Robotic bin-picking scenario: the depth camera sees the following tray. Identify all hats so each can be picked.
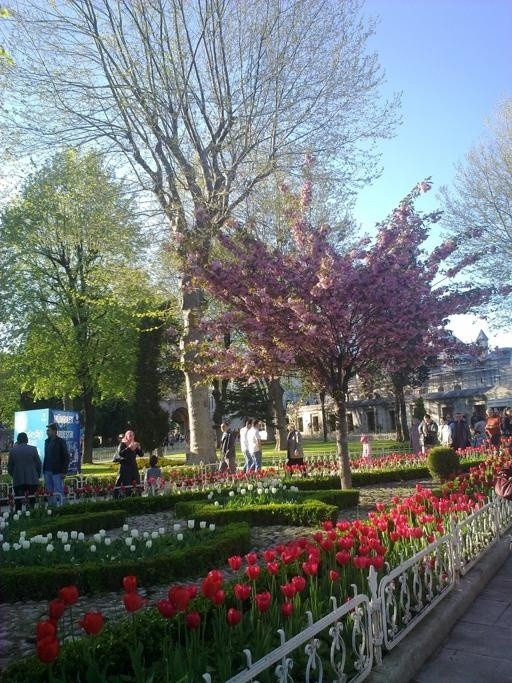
[45,423,57,429]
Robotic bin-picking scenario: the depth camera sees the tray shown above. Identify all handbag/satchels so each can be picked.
[112,450,128,463]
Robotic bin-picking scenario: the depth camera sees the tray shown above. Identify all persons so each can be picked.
[43,423,70,506]
[8,431,42,511]
[284,422,311,478]
[247,419,262,471]
[240,418,254,473]
[360,431,371,458]
[219,421,236,473]
[417,405,511,453]
[113,429,144,497]
